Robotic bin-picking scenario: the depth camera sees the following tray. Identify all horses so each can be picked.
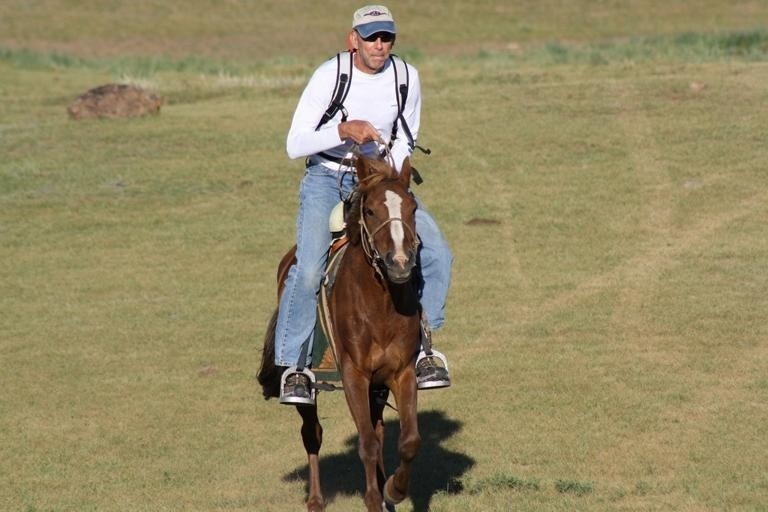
[256,155,423,512]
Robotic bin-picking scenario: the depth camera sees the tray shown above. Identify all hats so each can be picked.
[352,4,396,38]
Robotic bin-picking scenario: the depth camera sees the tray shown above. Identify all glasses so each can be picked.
[362,33,393,42]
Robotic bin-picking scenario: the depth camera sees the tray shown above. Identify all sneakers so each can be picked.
[415,358,448,381]
[285,374,311,397]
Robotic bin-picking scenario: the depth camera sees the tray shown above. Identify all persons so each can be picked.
[274,5,454,396]
